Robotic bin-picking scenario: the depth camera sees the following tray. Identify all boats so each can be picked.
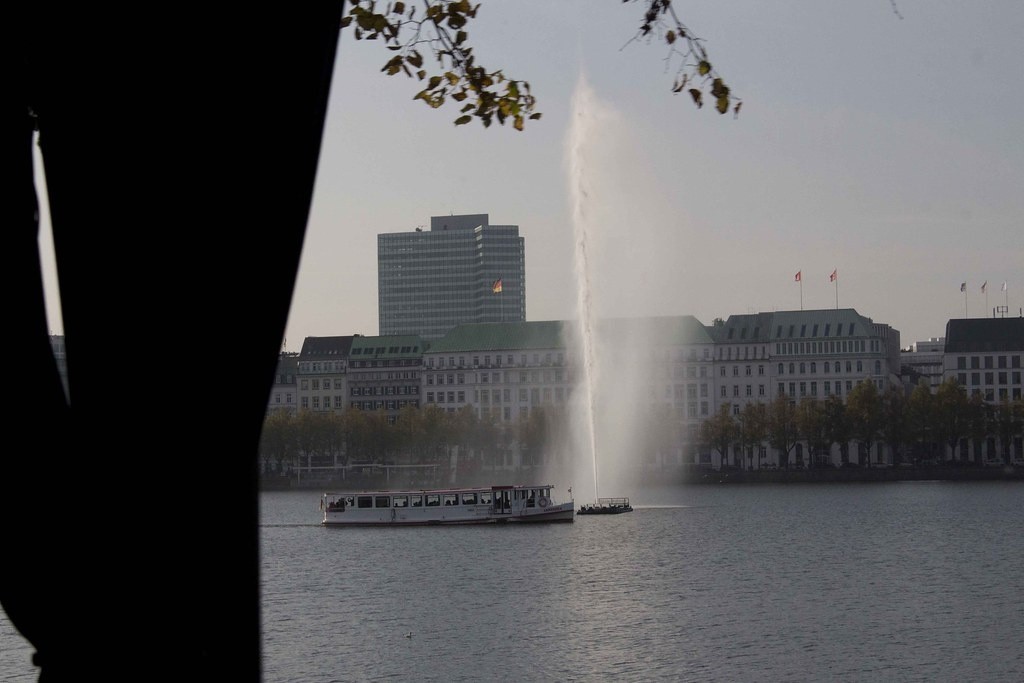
[321,484,575,527]
[577,497,634,516]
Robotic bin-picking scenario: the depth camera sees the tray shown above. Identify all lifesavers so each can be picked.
[538,498,548,507]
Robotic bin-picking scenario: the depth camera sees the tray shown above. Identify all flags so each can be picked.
[959,282,966,296]
[1001,281,1006,290]
[795,271,801,281]
[830,269,836,282]
[981,281,986,293]
[493,278,502,293]
